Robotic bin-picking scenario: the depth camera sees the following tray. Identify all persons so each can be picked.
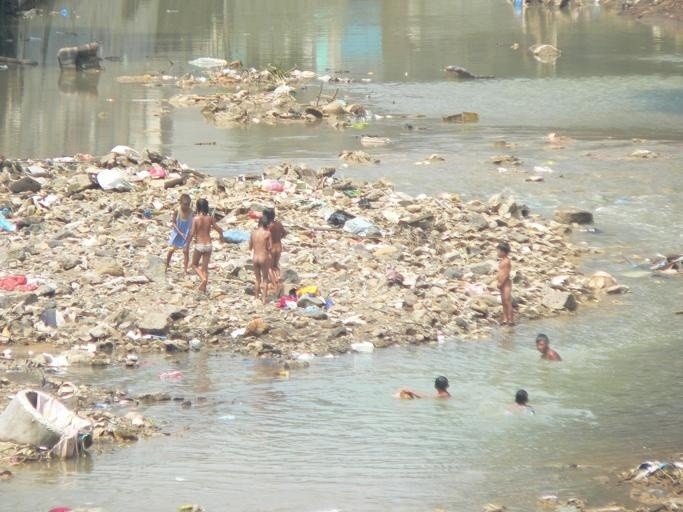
[494,240,516,330]
[261,207,289,288]
[163,194,196,277]
[185,198,224,293]
[534,333,562,363]
[400,376,452,402]
[247,215,273,301]
[510,387,533,411]
